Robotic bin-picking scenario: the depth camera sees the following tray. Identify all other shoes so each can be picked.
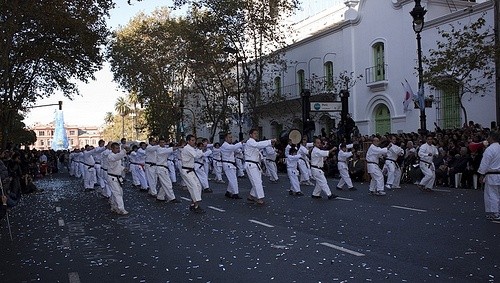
[132,184,163,201]
[337,186,342,190]
[385,185,391,189]
[312,195,322,199]
[86,183,100,192]
[350,187,357,191]
[190,204,206,214]
[328,194,337,200]
[225,191,242,199]
[368,190,386,195]
[102,193,129,214]
[290,190,304,196]
[392,186,401,189]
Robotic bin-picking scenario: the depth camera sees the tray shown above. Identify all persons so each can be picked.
[336,145,358,192]
[417,137,439,191]
[285,140,305,196]
[296,126,362,185]
[361,121,500,186]
[180,134,207,213]
[0,149,68,228]
[366,138,388,196]
[384,136,405,190]
[478,132,500,224]
[67,126,278,202]
[310,138,340,199]
[244,128,277,204]
[107,143,129,215]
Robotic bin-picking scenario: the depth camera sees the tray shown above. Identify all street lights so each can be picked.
[408,0,428,143]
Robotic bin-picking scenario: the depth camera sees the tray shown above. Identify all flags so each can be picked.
[402,79,415,113]
